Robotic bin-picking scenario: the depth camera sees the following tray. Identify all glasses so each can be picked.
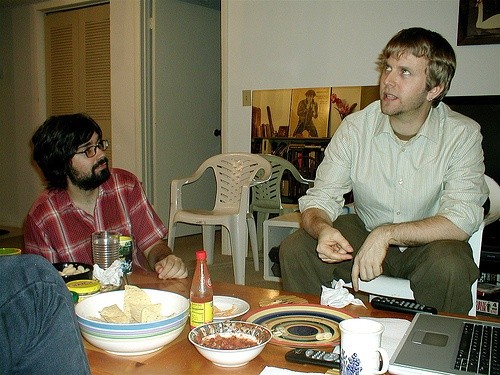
[75,139,109,158]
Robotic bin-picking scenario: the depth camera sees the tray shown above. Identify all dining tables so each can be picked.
[79,273,500,375]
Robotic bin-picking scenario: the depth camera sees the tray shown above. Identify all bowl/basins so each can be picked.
[65,279,100,296]
[74,288,190,355]
[51,261,93,282]
[188,321,273,368]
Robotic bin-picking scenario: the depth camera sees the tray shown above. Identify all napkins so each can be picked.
[320,285,368,308]
[94,260,125,287]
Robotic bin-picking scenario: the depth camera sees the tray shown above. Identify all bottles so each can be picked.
[190,251,213,331]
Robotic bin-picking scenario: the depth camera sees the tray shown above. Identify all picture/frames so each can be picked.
[457,0,500,46]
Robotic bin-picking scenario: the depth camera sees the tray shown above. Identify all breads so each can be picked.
[87,285,176,323]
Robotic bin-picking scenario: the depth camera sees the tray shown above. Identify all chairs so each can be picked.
[233,153,316,261]
[168,152,273,286]
[339,174,500,316]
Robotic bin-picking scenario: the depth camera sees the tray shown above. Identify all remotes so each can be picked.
[284,348,341,369]
[371,297,438,316]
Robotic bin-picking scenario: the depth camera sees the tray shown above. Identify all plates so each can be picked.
[240,304,359,350]
[211,295,251,321]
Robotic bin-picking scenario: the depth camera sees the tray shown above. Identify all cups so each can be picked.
[339,318,390,375]
[118,236,133,276]
[0,247,21,256]
[91,231,120,291]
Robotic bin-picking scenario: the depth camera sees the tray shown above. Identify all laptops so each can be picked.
[389,312,500,375]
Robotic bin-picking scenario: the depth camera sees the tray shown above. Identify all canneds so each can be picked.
[65,280,103,302]
[111,235,133,275]
[0,247,22,256]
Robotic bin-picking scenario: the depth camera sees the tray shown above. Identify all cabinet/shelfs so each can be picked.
[251,136,354,218]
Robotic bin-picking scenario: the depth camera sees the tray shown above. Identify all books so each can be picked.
[258,139,327,200]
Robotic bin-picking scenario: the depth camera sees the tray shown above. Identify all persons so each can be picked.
[0,254,93,375]
[279,28,490,315]
[24,113,190,279]
